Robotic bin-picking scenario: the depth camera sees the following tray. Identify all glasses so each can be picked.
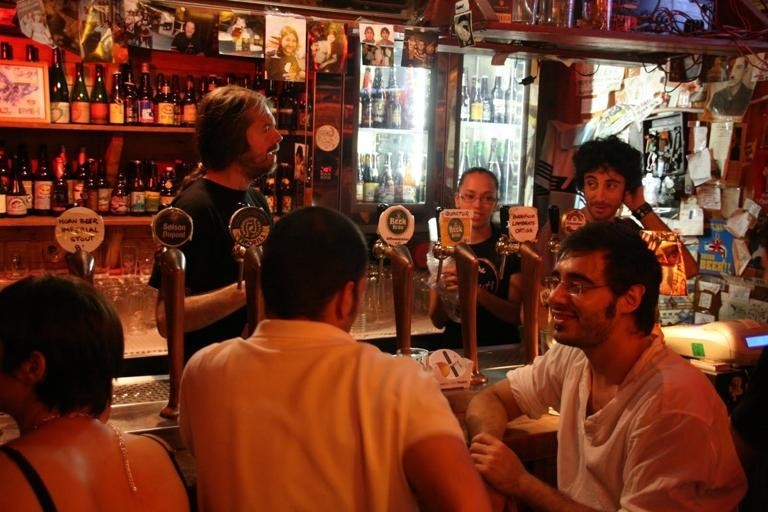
[543,276,609,297]
[457,187,497,205]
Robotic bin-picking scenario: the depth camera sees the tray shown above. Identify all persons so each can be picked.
[464,222,748,511]
[539,137,699,280]
[705,55,753,117]
[147,86,283,368]
[171,21,204,55]
[265,26,305,82]
[729,214,768,512]
[0,272,196,511]
[401,29,438,70]
[83,4,161,63]
[177,204,492,511]
[362,27,395,66]
[309,23,339,73]
[428,168,523,349]
[218,10,264,55]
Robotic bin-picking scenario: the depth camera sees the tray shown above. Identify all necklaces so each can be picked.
[33,411,139,494]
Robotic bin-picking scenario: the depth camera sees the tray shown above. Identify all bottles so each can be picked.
[1,38,302,131]
[353,58,526,206]
[0,140,299,221]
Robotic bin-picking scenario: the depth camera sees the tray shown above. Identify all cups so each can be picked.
[0,240,434,336]
[397,347,430,369]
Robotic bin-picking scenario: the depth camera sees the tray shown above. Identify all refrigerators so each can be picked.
[303,6,545,243]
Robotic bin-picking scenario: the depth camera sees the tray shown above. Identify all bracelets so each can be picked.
[631,203,652,222]
